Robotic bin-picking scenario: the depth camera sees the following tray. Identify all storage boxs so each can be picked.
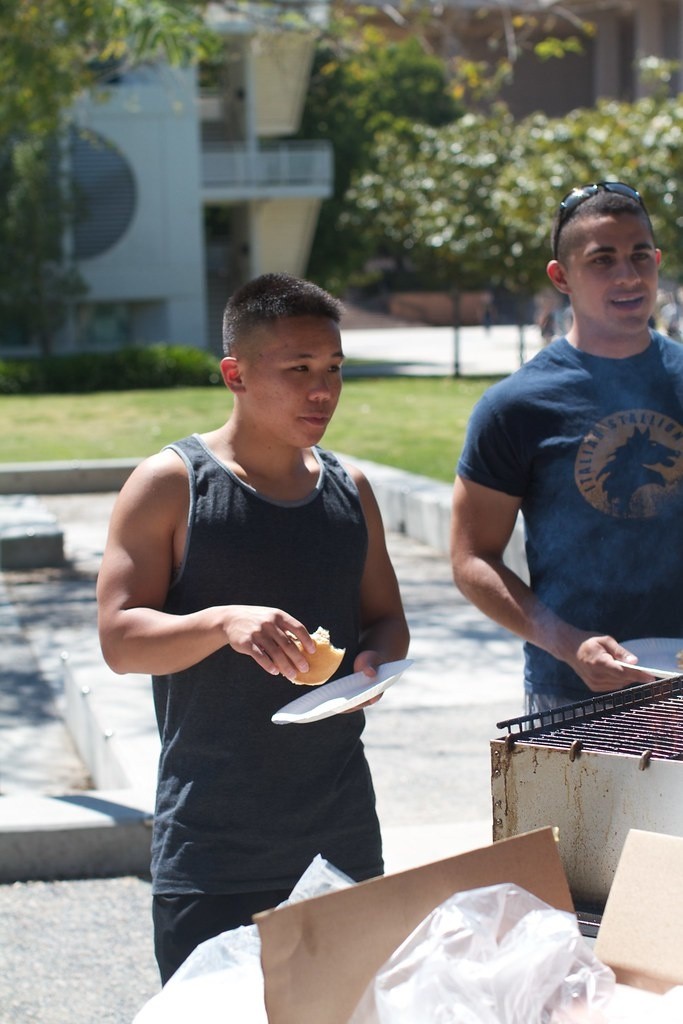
[252,825,683,1023]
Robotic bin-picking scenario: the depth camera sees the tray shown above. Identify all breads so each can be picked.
[283,625,346,686]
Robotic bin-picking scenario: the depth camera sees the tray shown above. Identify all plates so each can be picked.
[270,659,417,724]
[612,638,683,679]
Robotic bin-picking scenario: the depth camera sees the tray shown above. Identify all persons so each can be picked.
[537,296,556,345]
[660,292,683,341]
[97,275,411,985]
[451,185,682,732]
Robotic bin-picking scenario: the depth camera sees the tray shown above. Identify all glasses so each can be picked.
[553,182,649,259]
